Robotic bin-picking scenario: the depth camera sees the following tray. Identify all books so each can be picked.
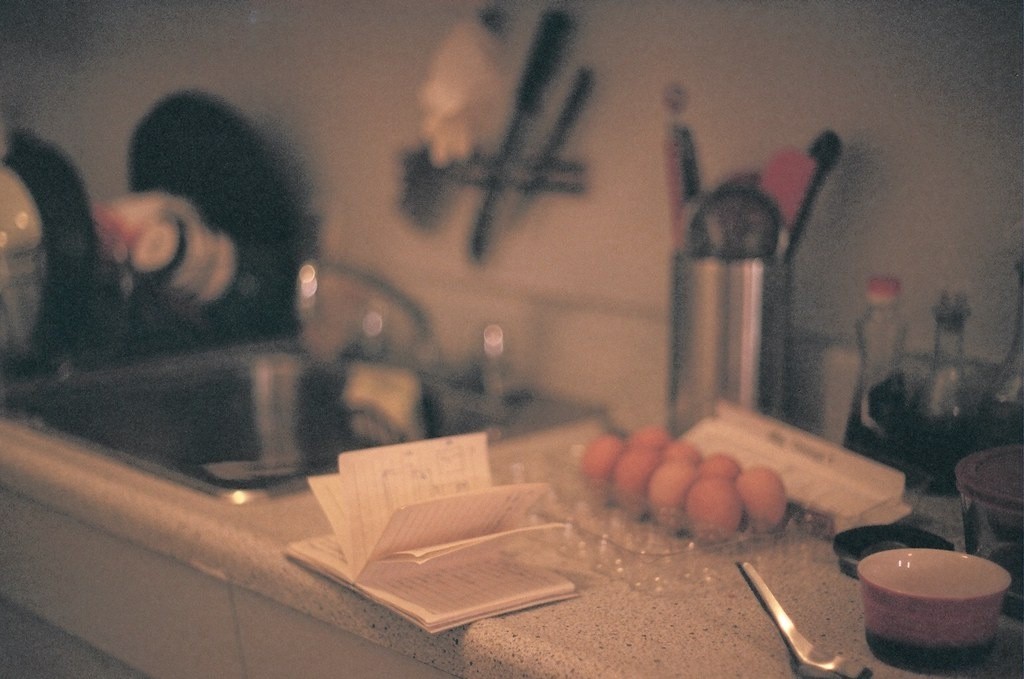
[283,431,582,633]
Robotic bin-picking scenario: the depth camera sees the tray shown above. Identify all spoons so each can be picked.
[736,560,873,679]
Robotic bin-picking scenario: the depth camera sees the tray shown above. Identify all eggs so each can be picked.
[581,425,786,544]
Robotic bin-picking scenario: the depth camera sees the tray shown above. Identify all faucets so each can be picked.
[311,253,438,379]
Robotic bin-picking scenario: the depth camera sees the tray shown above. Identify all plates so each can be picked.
[1,131,93,367]
[126,87,293,243]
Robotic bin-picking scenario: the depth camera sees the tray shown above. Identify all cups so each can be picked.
[670,247,793,439]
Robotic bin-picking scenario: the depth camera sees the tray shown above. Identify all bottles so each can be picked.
[859,259,1023,436]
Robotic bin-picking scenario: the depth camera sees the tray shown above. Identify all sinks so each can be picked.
[0,336,610,503]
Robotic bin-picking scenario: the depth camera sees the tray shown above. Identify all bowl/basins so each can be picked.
[856,547,1012,650]
[954,443,1024,615]
